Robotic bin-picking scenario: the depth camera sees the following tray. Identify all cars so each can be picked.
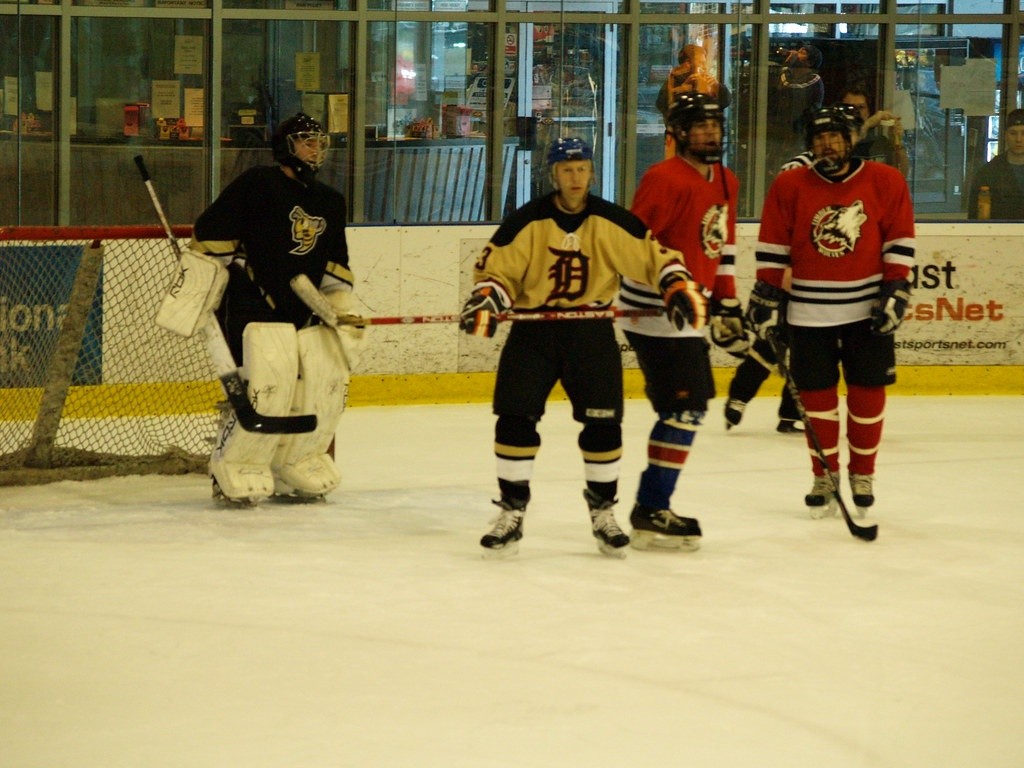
[649,64,674,82]
[918,68,964,126]
[398,21,468,33]
[390,54,417,106]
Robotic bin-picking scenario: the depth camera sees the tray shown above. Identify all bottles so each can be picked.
[977,187,991,219]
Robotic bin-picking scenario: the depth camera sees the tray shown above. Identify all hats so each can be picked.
[803,44,823,69]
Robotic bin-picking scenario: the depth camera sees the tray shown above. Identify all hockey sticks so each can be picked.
[292,271,665,328]
[135,153,318,434]
[766,328,879,540]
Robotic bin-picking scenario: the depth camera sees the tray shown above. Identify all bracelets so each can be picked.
[889,142,906,151]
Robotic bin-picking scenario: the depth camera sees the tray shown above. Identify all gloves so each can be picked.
[708,298,758,353]
[746,281,789,344]
[867,281,912,339]
[658,274,712,332]
[458,286,504,339]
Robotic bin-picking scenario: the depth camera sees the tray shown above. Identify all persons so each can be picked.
[460,136,710,549]
[655,44,733,160]
[723,46,909,428]
[749,110,915,506]
[966,109,1024,222]
[615,91,755,536]
[188,113,366,501]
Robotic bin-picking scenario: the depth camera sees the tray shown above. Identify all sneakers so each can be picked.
[846,469,875,517]
[630,500,703,553]
[777,419,806,436]
[479,498,523,559]
[724,396,746,429]
[211,477,258,509]
[804,473,840,518]
[582,488,630,560]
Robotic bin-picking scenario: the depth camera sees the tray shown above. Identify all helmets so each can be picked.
[272,110,331,188]
[664,89,731,164]
[805,107,853,176]
[547,138,598,192]
